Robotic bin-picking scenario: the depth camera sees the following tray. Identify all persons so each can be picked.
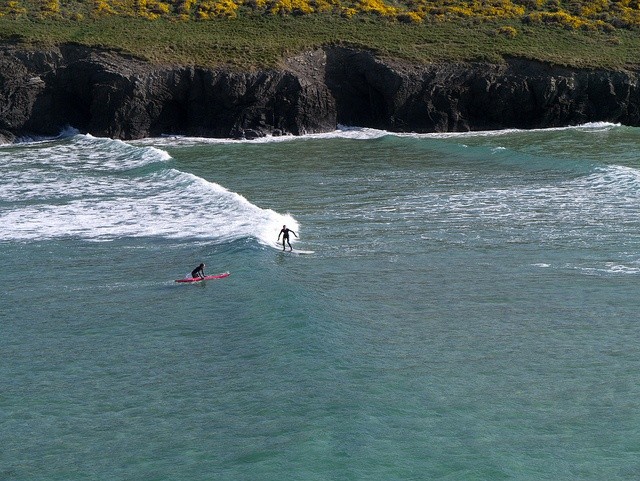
[277,225,297,251]
[192,263,208,279]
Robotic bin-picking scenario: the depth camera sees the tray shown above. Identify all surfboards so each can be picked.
[284,247,315,254]
[175,273,229,282]
